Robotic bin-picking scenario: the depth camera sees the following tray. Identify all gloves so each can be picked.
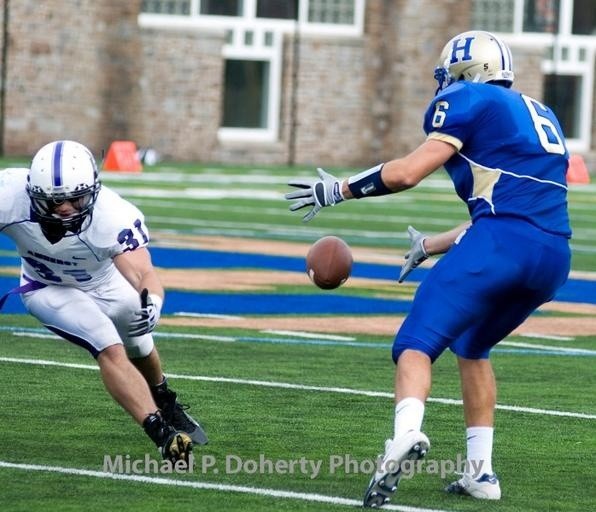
[398,224,431,286]
[284,167,346,224]
[127,286,163,338]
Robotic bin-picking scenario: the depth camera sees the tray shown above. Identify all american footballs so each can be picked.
[305,235,353,290]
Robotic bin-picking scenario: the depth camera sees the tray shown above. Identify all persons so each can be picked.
[285,30,572,509]
[0,140,208,472]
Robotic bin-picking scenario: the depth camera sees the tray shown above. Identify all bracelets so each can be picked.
[149,294,163,309]
[345,164,392,199]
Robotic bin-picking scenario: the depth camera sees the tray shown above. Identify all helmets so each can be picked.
[25,140,101,244]
[433,28,514,95]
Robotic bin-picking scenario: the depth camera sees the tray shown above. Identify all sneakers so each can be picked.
[361,428,431,509]
[156,388,208,445]
[142,411,194,470]
[443,471,503,500]
